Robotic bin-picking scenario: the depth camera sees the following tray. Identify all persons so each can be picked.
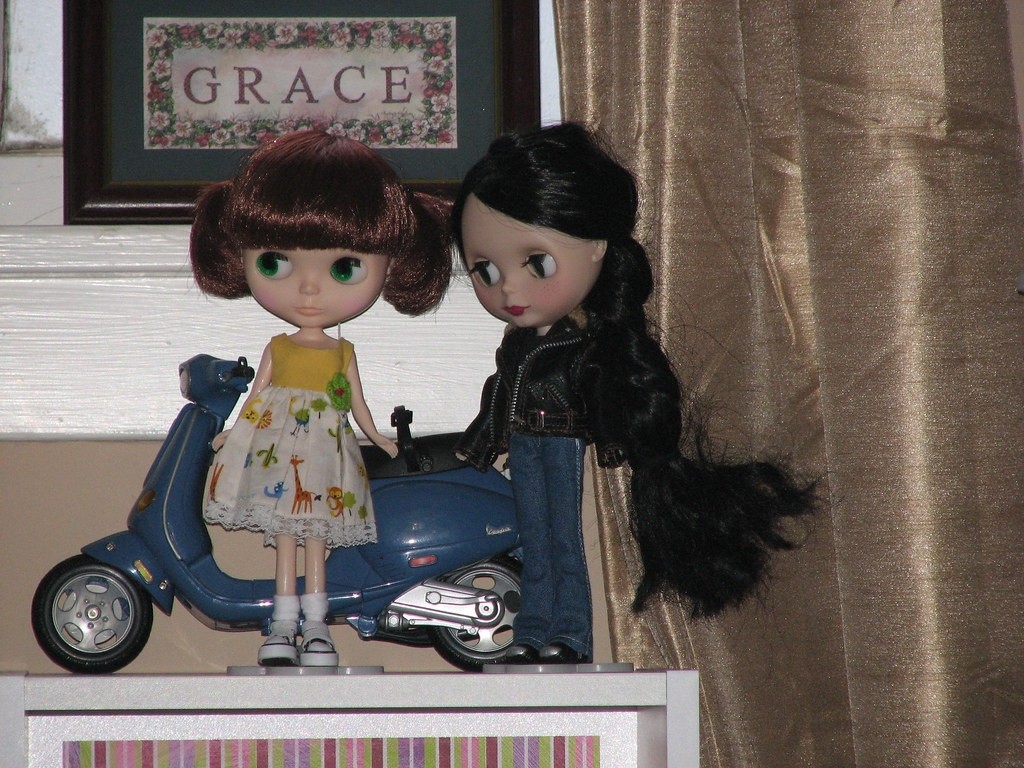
[191,134,456,664]
[449,122,828,664]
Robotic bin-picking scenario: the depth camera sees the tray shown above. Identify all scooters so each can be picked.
[30,353,497,675]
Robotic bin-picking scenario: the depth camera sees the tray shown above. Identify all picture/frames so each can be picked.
[62,0,541,224]
